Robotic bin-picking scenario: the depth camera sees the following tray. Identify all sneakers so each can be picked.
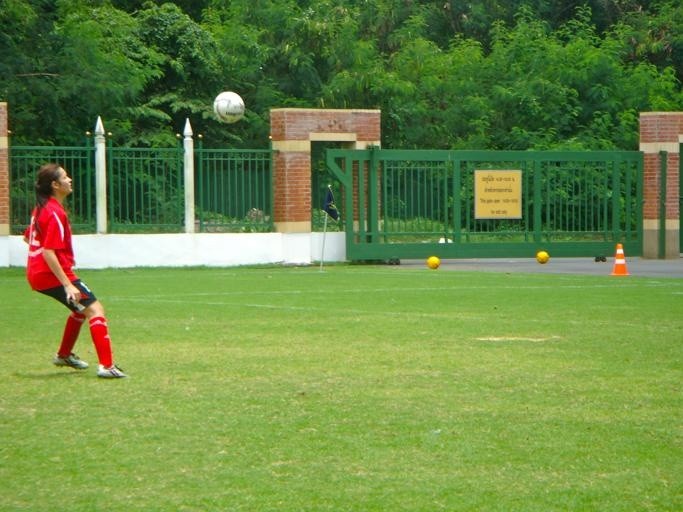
[95,363,129,378]
[52,351,90,369]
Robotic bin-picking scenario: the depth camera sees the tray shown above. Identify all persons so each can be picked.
[20,162,129,378]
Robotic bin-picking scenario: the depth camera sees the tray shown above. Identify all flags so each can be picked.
[321,186,339,221]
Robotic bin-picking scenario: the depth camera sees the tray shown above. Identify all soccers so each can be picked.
[213,91,245,124]
[426,256,440,268]
[537,251,550,264]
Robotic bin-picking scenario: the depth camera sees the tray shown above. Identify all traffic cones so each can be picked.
[608,243,632,276]
